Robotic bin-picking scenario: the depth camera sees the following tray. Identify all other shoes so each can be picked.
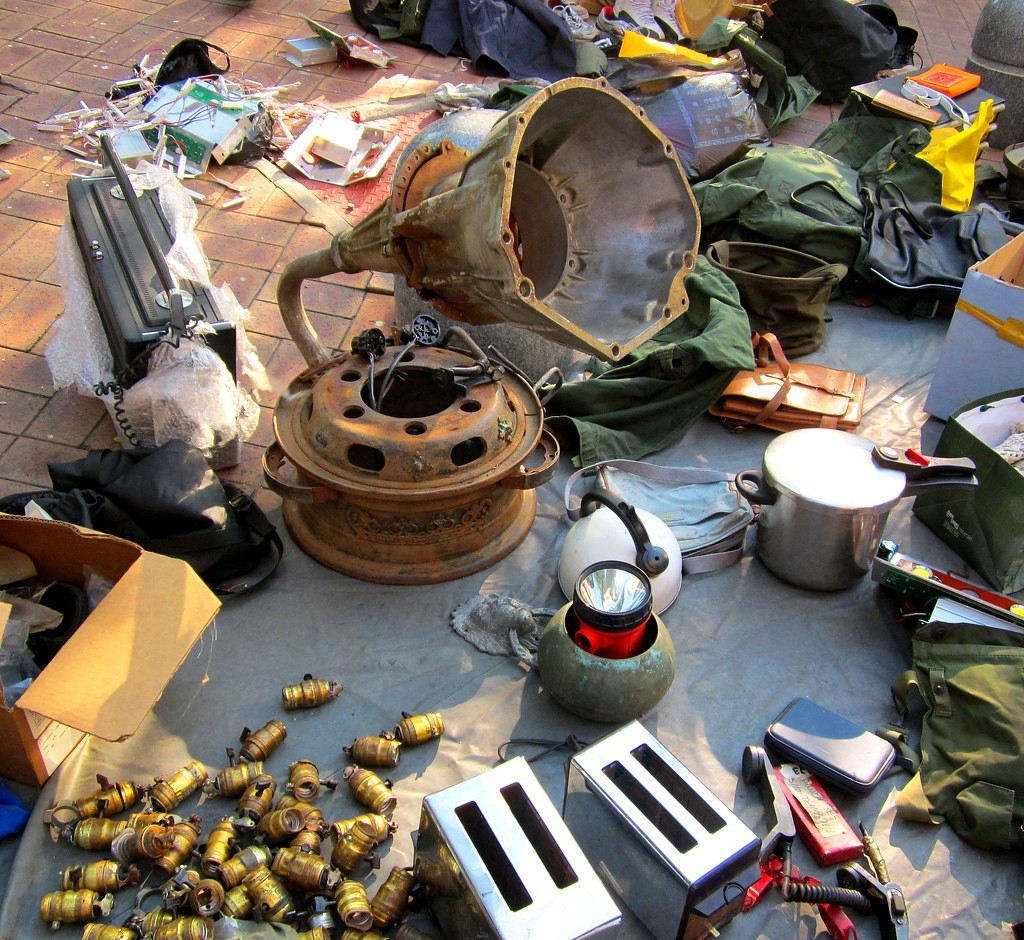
[889,25,924,70]
[561,0,590,20]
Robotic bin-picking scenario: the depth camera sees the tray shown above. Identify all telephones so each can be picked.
[740,741,796,862]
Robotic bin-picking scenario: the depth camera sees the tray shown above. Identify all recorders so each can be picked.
[67,135,242,472]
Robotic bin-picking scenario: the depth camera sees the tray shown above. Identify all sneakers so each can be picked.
[544,0,598,41]
[651,0,691,48]
[613,0,665,40]
[595,5,643,42]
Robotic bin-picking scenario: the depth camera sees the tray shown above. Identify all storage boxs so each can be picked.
[312,113,365,168]
[104,128,153,168]
[923,225,1023,421]
[0,514,223,789]
[284,36,339,69]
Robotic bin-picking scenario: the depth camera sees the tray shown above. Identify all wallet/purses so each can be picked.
[763,696,897,797]
[771,762,865,867]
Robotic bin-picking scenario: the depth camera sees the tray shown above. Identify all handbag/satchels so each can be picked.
[789,178,1007,320]
[706,238,849,361]
[603,54,775,186]
[911,386,1024,595]
[563,456,754,574]
[879,621,1024,853]
[691,15,821,137]
[709,331,870,434]
[1,439,284,599]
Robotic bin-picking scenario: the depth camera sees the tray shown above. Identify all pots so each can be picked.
[736,428,980,591]
